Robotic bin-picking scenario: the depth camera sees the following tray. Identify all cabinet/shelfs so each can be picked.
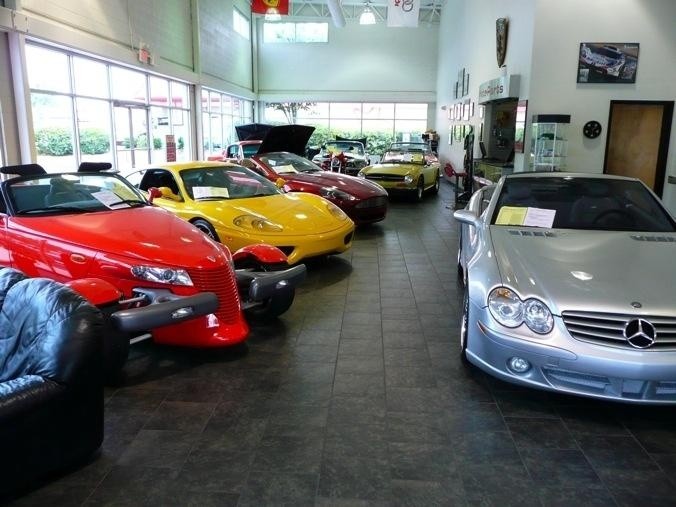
[528,113,571,172]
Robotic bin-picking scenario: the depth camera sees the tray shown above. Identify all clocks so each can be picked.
[583,121,602,138]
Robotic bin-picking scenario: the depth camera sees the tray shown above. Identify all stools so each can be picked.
[445,163,469,210]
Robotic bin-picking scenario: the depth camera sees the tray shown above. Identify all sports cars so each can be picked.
[451,167,676,405]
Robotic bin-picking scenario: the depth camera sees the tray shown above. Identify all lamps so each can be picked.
[360,0,378,26]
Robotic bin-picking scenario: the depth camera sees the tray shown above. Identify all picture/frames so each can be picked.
[576,42,639,83]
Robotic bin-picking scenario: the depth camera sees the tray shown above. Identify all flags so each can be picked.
[386,0,420,28]
[251,0,289,16]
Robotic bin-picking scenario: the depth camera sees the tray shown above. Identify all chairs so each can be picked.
[501,182,539,210]
[571,182,621,228]
[44,178,82,206]
